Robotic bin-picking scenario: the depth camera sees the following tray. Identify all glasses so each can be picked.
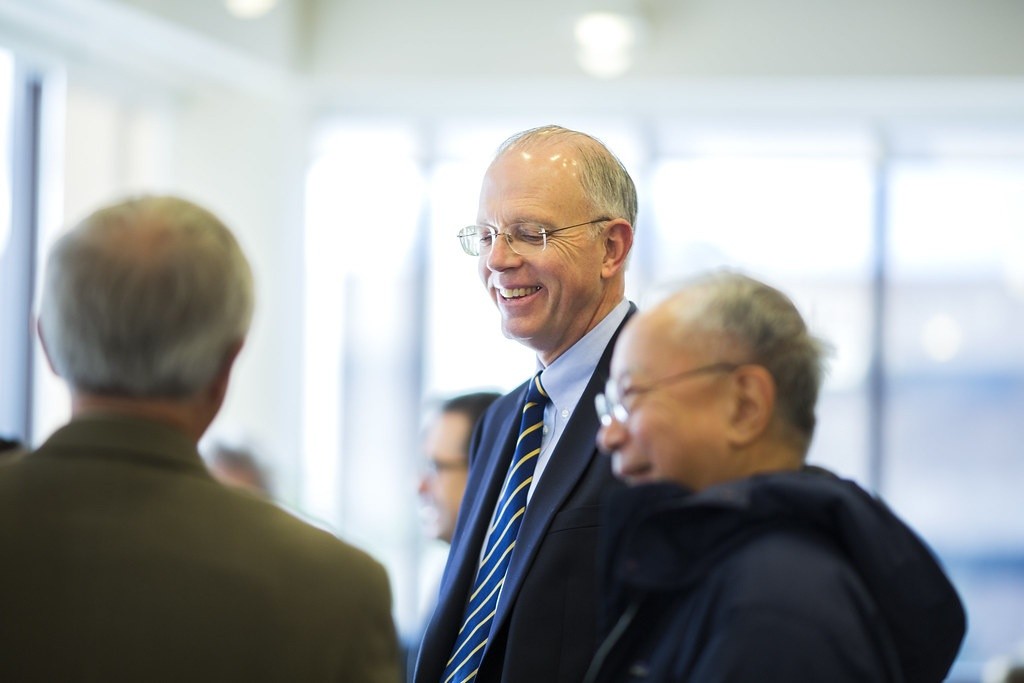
[594,364,740,425]
[458,217,610,261]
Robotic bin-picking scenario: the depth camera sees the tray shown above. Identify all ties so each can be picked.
[440,373,551,683]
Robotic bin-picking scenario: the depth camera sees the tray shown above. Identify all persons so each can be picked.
[417,389,513,548]
[596,270,968,683]
[205,448,278,510]
[403,128,648,683]
[0,199,409,683]
